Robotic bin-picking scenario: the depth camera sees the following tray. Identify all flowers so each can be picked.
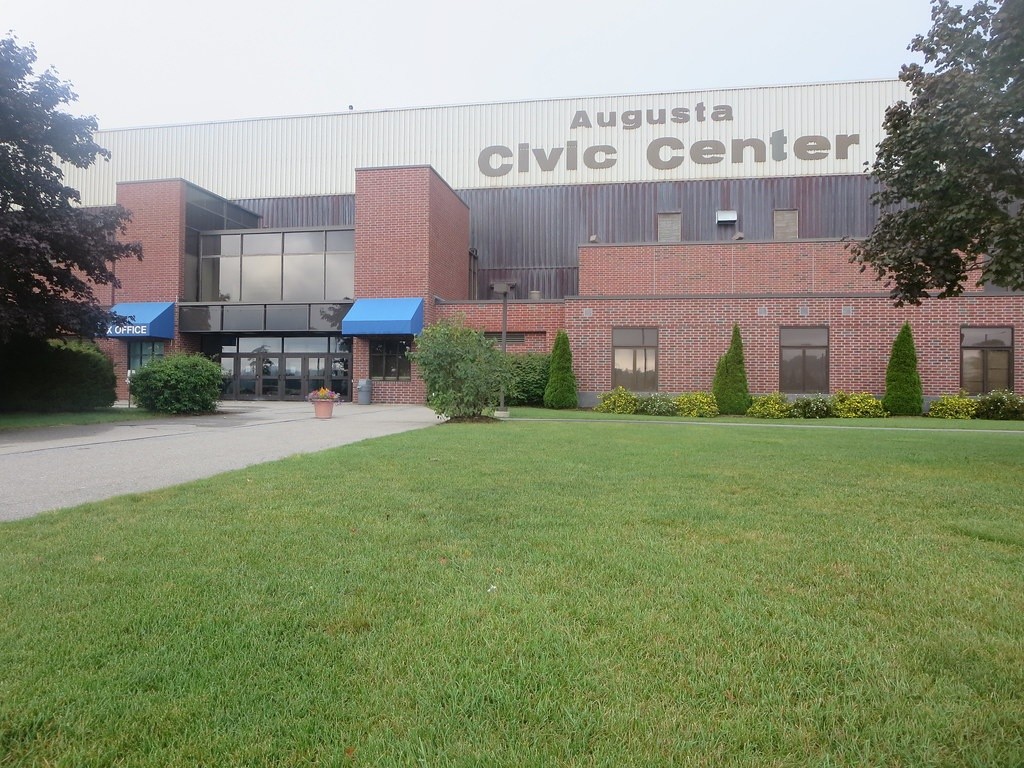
[306,387,344,407]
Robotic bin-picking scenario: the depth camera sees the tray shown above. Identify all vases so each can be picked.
[310,398,334,419]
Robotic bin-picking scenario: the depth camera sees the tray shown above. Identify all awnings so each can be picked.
[342,298,424,335]
[96,302,176,339]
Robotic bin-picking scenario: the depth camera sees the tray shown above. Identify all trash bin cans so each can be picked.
[356,379,372,405]
